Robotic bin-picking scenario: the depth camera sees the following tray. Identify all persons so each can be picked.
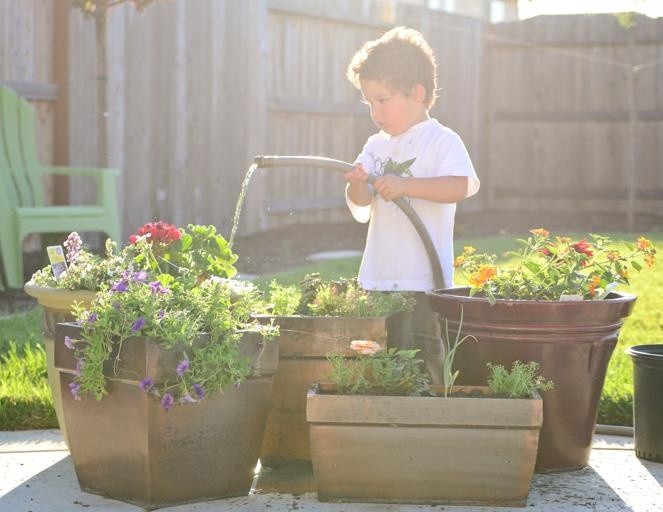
[346,27,481,387]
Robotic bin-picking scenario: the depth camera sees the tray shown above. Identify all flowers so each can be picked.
[452,228,657,303]
[259,272,418,318]
[63,271,280,409]
[322,304,557,397]
[28,218,238,287]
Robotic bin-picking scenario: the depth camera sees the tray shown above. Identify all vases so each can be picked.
[425,285,637,477]
[625,345,663,463]
[22,282,255,454]
[252,312,387,475]
[305,382,544,509]
[52,320,279,511]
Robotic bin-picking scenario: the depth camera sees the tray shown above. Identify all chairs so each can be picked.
[0,83,121,290]
